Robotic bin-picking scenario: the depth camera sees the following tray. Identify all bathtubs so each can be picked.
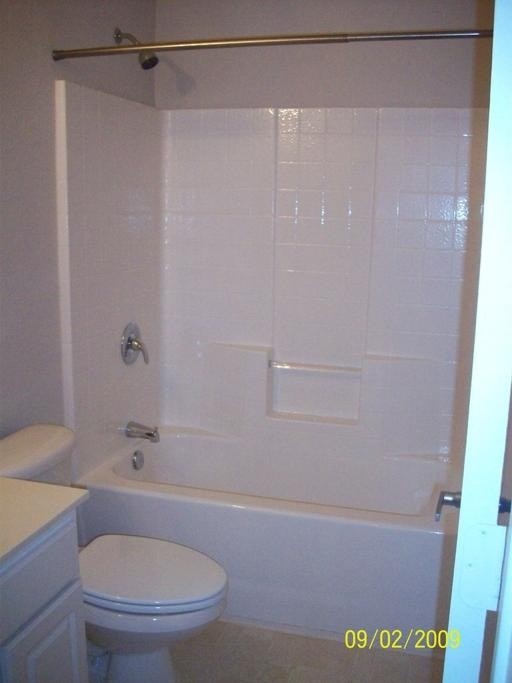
[70,425,464,662]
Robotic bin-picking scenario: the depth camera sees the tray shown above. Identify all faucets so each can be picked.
[124,421,160,443]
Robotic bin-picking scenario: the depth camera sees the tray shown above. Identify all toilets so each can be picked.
[0,421,227,681]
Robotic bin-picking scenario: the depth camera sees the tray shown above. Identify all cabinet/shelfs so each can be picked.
[0,522,93,683]
